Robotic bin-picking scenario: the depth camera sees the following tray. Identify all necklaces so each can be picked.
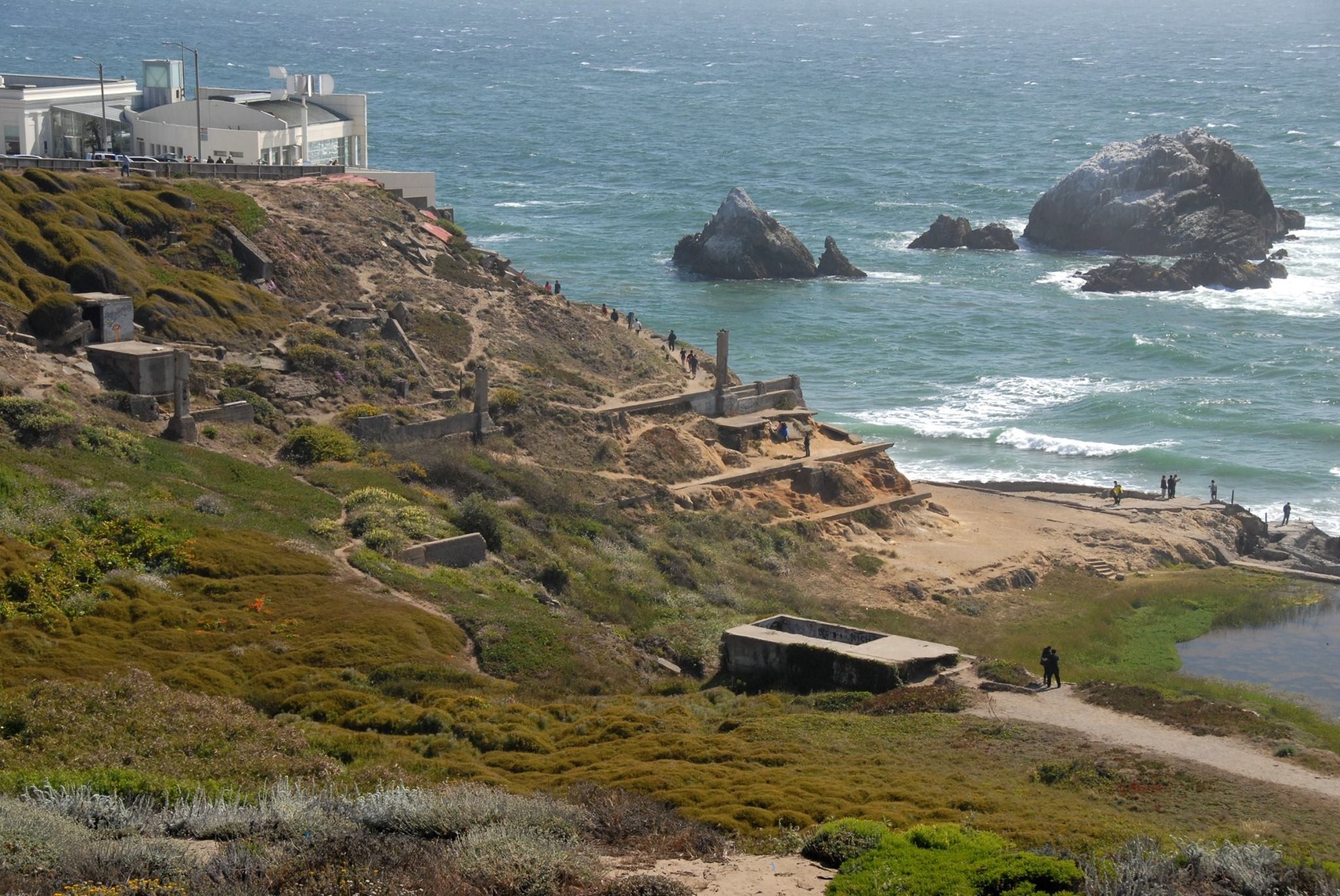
[632,318,641,335]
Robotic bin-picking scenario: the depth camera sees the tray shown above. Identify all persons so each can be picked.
[625,312,636,330]
[1167,474,1180,499]
[1040,645,1062,689]
[327,159,339,166]
[667,330,676,351]
[600,304,608,318]
[691,354,698,380]
[1112,481,1123,508]
[544,281,552,292]
[686,350,694,373]
[1210,480,1217,501]
[1161,475,1167,497]
[120,153,132,177]
[555,280,561,294]
[183,155,234,164]
[680,348,687,367]
[776,419,790,442]
[1282,502,1291,526]
[610,309,619,324]
[803,432,811,458]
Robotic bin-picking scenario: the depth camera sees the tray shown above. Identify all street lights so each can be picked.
[72,56,107,153]
[162,41,201,164]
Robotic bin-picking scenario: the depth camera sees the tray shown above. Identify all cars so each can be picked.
[0,154,42,158]
[92,152,179,162]
